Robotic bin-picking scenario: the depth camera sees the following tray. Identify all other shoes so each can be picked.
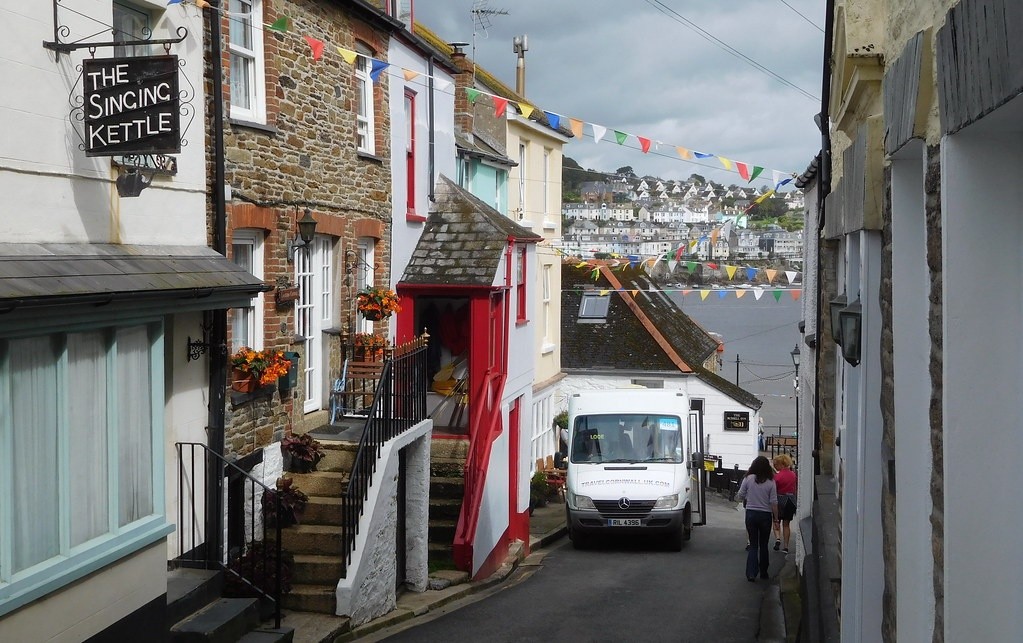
[782,548,788,553]
[761,573,769,578]
[748,576,755,582]
[745,543,750,549]
[774,540,781,550]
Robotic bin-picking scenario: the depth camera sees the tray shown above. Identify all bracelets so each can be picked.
[774,520,779,523]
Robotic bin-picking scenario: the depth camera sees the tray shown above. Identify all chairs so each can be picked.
[537,455,567,504]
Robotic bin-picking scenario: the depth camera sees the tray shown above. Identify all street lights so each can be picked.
[790,342,800,465]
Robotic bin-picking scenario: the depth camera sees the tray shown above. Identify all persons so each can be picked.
[737,456,780,583]
[773,454,798,554]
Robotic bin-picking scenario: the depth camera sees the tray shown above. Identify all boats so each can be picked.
[666,281,802,289]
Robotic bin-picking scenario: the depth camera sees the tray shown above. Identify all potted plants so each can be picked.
[261,475,310,530]
[281,432,326,473]
[225,538,294,619]
[529,472,549,516]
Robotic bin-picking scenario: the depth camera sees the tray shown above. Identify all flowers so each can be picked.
[230,346,292,387]
[352,332,390,358]
[355,285,402,321]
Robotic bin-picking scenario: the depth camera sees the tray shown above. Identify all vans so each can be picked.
[553,388,703,551]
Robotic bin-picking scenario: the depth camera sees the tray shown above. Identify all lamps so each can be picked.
[837,290,862,367]
[827,289,847,347]
[287,199,318,263]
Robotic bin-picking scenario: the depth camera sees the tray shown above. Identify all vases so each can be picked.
[365,310,378,320]
[232,368,256,393]
[354,356,372,363]
